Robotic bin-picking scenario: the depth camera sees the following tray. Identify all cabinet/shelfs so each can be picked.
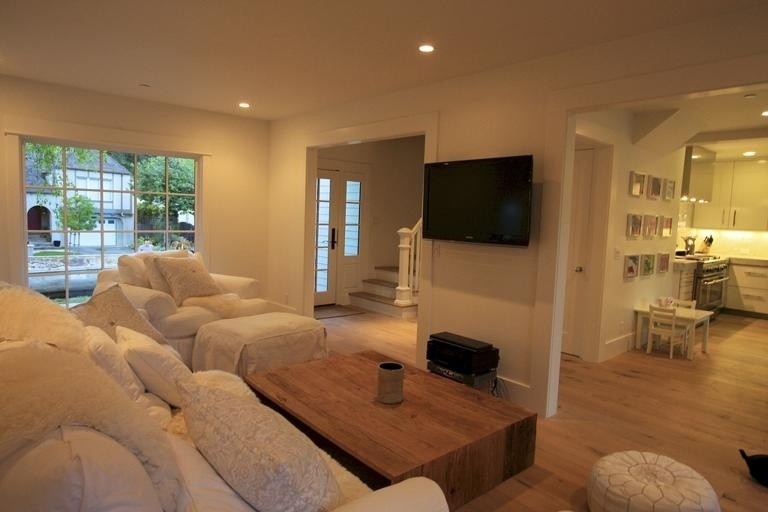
[723,261,768,315]
[688,157,768,231]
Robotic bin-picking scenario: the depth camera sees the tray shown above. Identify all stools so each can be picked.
[191,310,329,378]
[584,448,720,512]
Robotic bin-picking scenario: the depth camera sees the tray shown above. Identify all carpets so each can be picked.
[315,304,366,320]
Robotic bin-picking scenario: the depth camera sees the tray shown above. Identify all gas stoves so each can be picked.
[680,254,728,277]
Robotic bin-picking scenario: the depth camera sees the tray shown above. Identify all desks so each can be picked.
[633,306,713,361]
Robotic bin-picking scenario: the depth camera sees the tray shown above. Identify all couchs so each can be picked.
[0,278,450,512]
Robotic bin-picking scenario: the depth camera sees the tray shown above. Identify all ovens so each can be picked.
[699,277,726,313]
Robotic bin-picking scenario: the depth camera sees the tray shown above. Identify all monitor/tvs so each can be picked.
[422,154,533,246]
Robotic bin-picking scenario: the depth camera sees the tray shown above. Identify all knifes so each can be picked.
[704,235,714,246]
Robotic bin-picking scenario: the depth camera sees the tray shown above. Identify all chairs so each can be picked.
[674,298,697,350]
[646,305,686,359]
[90,265,269,371]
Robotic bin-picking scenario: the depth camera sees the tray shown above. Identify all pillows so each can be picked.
[83,323,175,433]
[112,322,193,406]
[65,281,170,346]
[138,249,189,293]
[175,366,344,511]
[152,254,219,302]
[116,254,153,288]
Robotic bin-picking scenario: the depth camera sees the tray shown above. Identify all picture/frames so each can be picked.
[620,169,678,280]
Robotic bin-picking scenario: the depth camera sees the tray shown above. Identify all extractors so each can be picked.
[679,144,716,205]
